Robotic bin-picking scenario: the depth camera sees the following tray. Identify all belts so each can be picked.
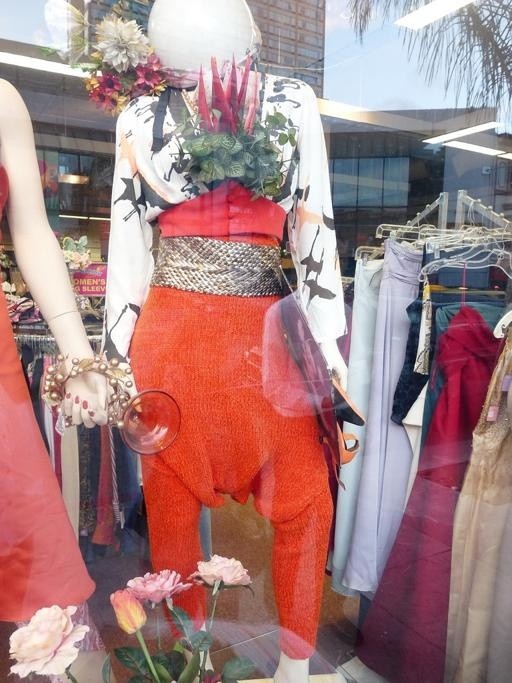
[149,236,285,298]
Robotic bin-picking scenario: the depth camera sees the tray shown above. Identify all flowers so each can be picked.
[7,543,261,683]
[40,0,175,112]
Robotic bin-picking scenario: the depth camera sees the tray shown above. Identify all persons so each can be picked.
[0,78,110,682]
[103,0,352,681]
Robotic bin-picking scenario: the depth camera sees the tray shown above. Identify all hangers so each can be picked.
[377,193,511,285]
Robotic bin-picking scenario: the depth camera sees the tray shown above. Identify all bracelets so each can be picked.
[42,352,134,429]
